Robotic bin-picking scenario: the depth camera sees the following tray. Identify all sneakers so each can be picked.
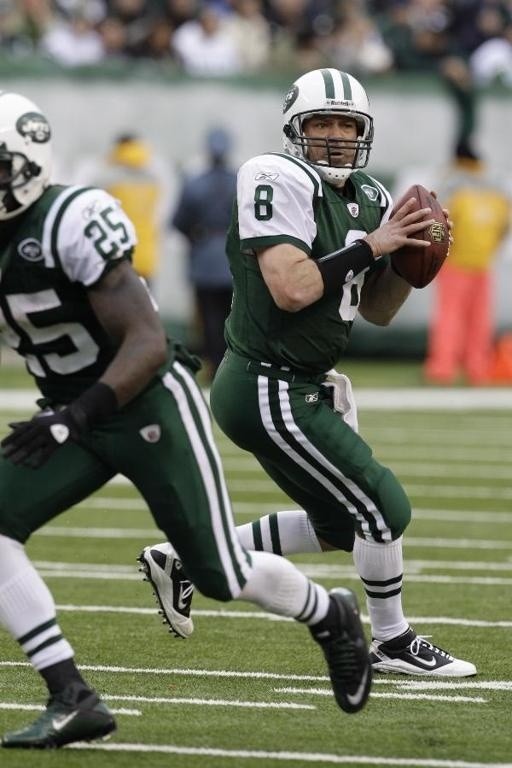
[135,541,195,638]
[320,585,372,714]
[368,627,480,677]
[3,688,118,749]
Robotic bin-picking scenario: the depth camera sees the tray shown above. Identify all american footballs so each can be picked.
[389,184,447,287]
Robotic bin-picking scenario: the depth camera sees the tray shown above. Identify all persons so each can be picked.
[0,87,378,752]
[168,124,242,384]
[416,152,510,389]
[72,130,181,303]
[132,65,484,683]
[0,0,512,170]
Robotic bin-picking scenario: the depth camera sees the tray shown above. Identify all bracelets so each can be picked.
[314,237,377,298]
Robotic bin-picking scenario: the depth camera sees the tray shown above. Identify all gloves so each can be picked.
[1,408,83,464]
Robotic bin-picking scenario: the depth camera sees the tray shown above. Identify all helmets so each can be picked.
[0,90,54,220]
[281,67,375,190]
